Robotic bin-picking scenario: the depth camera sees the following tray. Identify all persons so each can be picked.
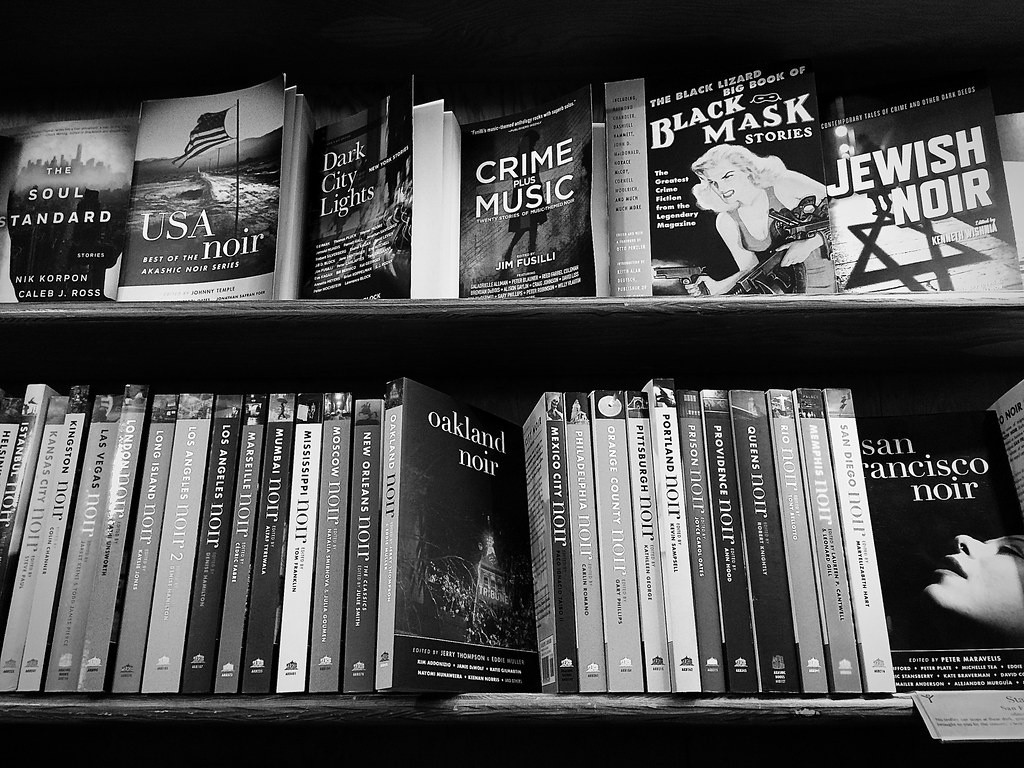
[683,143,834,297]
[503,128,548,275]
[922,533,1024,649]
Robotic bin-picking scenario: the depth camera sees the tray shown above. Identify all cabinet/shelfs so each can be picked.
[0,290,1024,721]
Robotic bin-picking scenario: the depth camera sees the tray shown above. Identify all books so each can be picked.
[0,377,1024,698]
[0,52,1024,306]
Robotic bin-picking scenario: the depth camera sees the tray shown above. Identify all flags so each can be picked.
[171,106,233,168]
